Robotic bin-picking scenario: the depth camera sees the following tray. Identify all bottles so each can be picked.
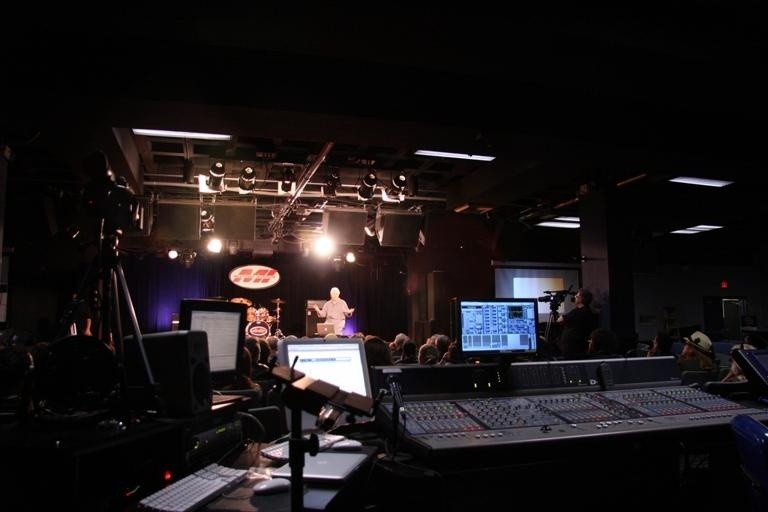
[683,331,713,353]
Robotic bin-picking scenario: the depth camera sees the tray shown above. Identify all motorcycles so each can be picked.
[544,310,560,343]
[54,260,158,386]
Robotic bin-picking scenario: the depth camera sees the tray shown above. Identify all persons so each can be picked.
[223,330,457,394]
[681,332,713,371]
[720,343,754,382]
[646,334,675,355]
[587,327,620,359]
[555,289,593,361]
[311,288,354,335]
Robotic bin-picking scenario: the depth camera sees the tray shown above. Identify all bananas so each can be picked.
[140,463,248,512]
[260,434,346,462]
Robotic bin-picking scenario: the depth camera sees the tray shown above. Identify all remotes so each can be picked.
[332,439,362,450]
[254,478,291,495]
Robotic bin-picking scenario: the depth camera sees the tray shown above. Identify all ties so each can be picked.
[453,297,539,362]
[732,348,768,405]
[278,339,375,432]
[179,298,247,405]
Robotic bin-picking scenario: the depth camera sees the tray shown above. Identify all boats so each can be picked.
[158,199,200,241]
[324,207,366,246]
[215,202,256,241]
[375,210,420,247]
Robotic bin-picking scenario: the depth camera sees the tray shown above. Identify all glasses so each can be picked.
[206,161,408,202]
